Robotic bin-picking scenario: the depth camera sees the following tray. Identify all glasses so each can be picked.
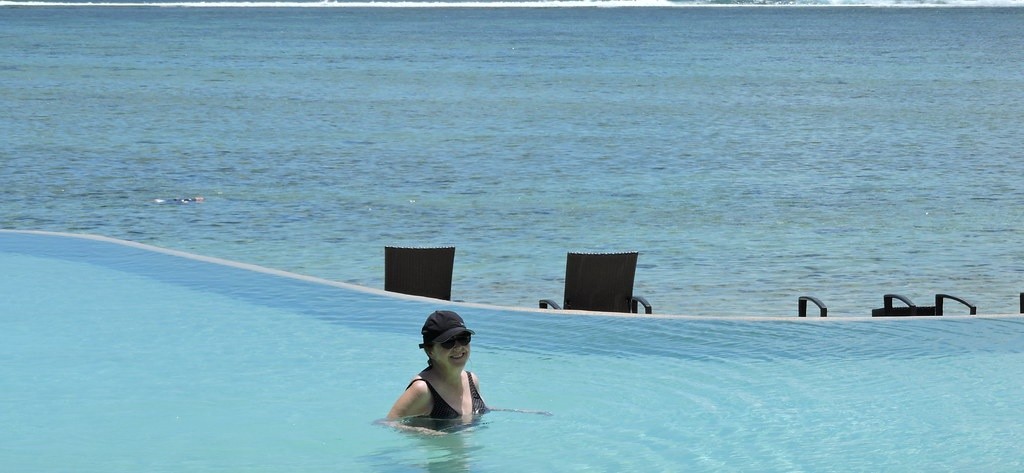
[441,330,472,349]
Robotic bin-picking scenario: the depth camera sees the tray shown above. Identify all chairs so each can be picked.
[539,251,652,315]
[385,245,463,302]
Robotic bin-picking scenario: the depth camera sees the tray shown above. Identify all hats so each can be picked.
[419,310,475,348]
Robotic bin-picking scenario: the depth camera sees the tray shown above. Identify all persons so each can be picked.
[376,310,553,439]
